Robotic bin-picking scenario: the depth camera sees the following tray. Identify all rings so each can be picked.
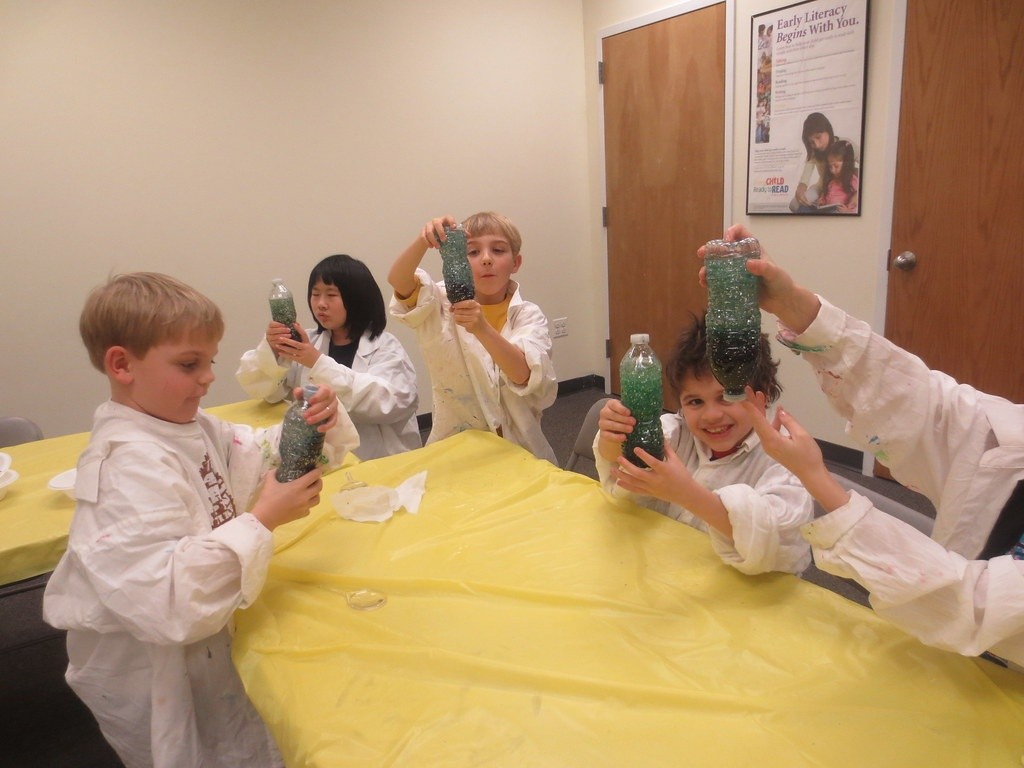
[327,407,334,413]
[293,349,297,356]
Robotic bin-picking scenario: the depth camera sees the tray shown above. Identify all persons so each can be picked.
[42,272,360,768]
[235,254,422,461]
[387,212,559,467]
[756,25,773,143]
[593,308,814,576]
[696,223,1024,657]
[788,113,858,213]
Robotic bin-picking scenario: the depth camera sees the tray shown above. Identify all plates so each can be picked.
[0,452,20,501]
[47,468,78,502]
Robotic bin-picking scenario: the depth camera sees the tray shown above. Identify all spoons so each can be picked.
[340,471,366,491]
[318,585,387,612]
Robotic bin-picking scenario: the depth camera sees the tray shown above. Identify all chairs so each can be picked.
[802,473,934,613]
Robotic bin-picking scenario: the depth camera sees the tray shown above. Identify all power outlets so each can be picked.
[552,316,570,339]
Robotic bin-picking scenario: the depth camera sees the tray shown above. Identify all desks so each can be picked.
[226,427,1024,768]
[1,398,361,596]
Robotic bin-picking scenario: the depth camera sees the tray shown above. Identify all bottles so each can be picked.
[436,222,475,304]
[269,278,302,343]
[274,385,326,483]
[618,333,665,469]
[704,237,761,403]
